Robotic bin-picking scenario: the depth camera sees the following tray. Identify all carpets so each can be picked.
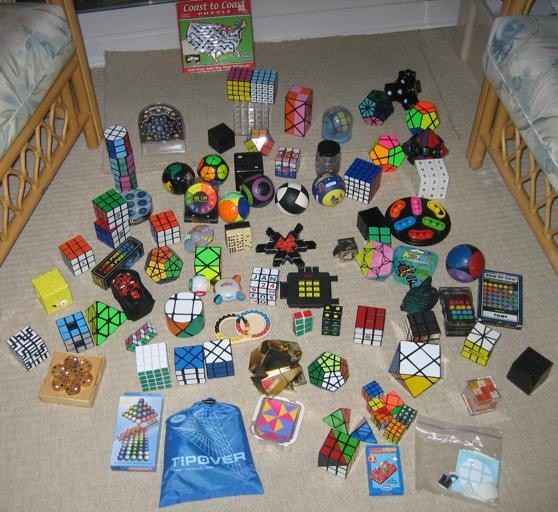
[101,29,462,173]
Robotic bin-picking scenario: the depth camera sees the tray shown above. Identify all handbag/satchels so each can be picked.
[159,397,264,507]
